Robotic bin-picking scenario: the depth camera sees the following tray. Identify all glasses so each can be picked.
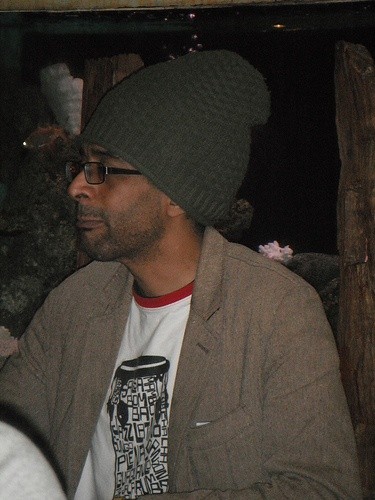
[64,159,145,185]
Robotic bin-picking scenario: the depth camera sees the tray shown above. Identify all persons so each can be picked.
[1,51,364,500]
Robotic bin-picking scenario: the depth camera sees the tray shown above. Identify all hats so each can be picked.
[81,51,271,227]
